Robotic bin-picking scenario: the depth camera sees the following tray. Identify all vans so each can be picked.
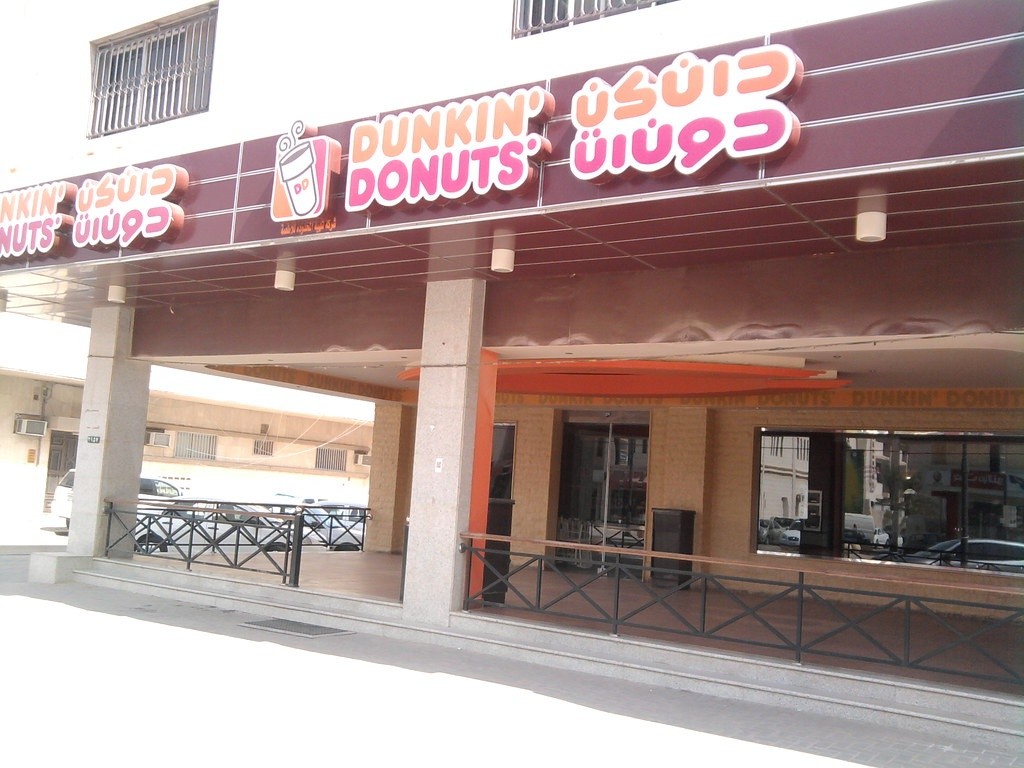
[898,536,1024,573]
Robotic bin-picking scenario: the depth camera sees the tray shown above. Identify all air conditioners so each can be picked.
[354,453,372,467]
[14,419,48,437]
[145,432,170,448]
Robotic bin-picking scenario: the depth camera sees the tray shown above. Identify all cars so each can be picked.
[875,528,889,548]
[759,516,801,546]
[574,504,644,563]
[134,493,368,552]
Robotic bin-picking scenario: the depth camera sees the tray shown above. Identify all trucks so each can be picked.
[844,513,874,543]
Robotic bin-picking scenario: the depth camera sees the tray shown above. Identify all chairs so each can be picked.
[226,514,234,521]
[350,509,360,521]
[558,518,593,570]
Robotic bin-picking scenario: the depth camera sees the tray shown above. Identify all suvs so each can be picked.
[50,468,185,530]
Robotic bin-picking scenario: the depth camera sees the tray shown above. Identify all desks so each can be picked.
[593,524,646,532]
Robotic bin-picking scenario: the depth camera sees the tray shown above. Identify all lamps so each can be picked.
[856,212,886,243]
[108,285,126,303]
[491,249,515,273]
[274,269,295,292]
